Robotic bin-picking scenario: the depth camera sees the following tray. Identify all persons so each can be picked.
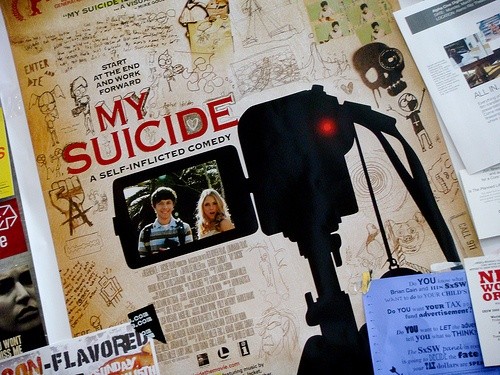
[474,63,489,84]
[136,187,193,259]
[449,49,464,64]
[194,190,232,241]
[1,263,46,353]
[327,20,344,41]
[370,21,388,41]
[488,22,498,36]
[318,2,335,23]
[359,3,377,24]
